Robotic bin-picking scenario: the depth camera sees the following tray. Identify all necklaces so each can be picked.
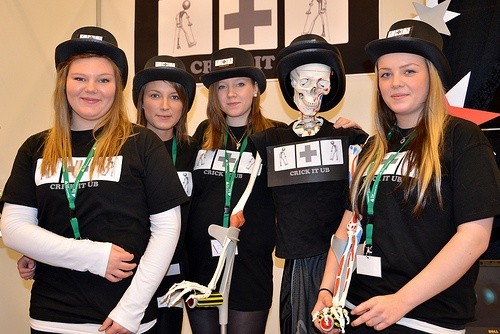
[395,125,418,144]
[224,116,248,150]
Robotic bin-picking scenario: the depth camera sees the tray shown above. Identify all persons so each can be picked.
[178,48,361,334]
[310,18,500,334]
[0,26,188,334]
[18,55,196,334]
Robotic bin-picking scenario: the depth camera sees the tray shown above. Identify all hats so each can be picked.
[276,34,346,112]
[133,55,196,112]
[201,47,267,96]
[55,26,128,92]
[364,19,451,73]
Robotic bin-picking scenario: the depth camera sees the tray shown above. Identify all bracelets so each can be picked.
[317,288,333,299]
